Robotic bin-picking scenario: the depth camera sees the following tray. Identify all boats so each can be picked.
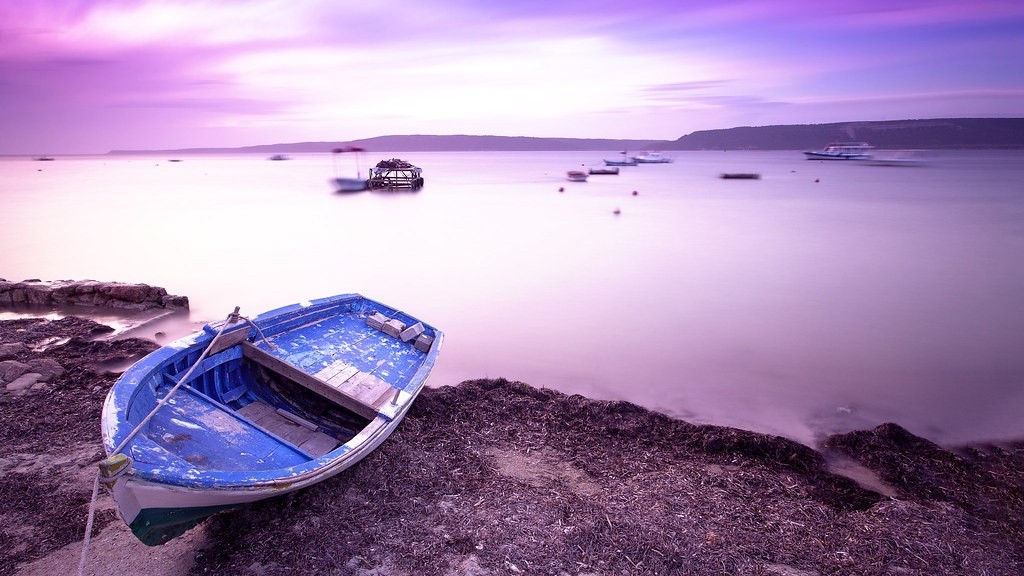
[632,152,671,163]
[98,293,446,546]
[603,158,637,166]
[722,172,759,180]
[330,178,370,192]
[802,142,874,160]
[590,167,619,175]
[567,170,589,183]
[271,154,289,160]
